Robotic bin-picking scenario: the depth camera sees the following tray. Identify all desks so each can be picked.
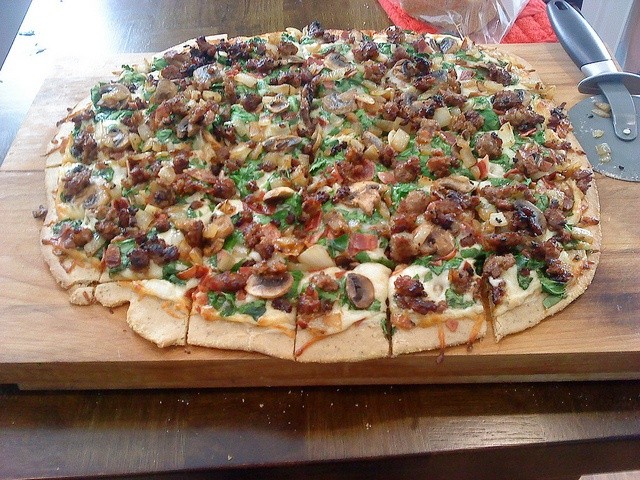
[1,0,640,480]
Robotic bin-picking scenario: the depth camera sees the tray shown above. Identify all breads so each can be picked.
[399,1,497,34]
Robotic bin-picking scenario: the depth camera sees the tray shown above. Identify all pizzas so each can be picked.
[39,22,603,361]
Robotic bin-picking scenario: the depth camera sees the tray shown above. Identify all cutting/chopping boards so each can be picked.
[1,40,638,371]
